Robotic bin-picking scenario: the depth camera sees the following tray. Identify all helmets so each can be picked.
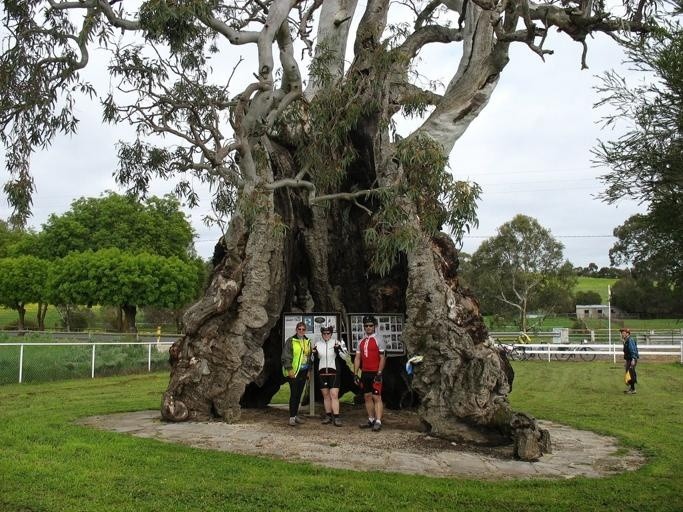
[320,324,333,333]
[620,328,630,334]
[363,315,377,324]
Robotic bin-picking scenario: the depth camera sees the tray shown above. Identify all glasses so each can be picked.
[365,325,373,327]
[297,328,306,331]
[323,333,330,335]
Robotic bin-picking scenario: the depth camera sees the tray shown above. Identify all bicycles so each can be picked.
[496,338,551,361]
[555,338,595,361]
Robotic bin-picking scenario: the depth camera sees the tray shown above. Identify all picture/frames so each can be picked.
[345,313,407,358]
[282,311,342,353]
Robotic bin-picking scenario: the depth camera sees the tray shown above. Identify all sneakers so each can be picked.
[359,418,382,432]
[322,415,342,427]
[624,388,636,394]
[289,415,305,426]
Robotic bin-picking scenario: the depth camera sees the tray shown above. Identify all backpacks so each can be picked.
[625,367,636,386]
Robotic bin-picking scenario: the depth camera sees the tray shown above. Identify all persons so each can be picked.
[620,327,639,395]
[309,322,349,427]
[351,314,387,432]
[280,321,313,426]
